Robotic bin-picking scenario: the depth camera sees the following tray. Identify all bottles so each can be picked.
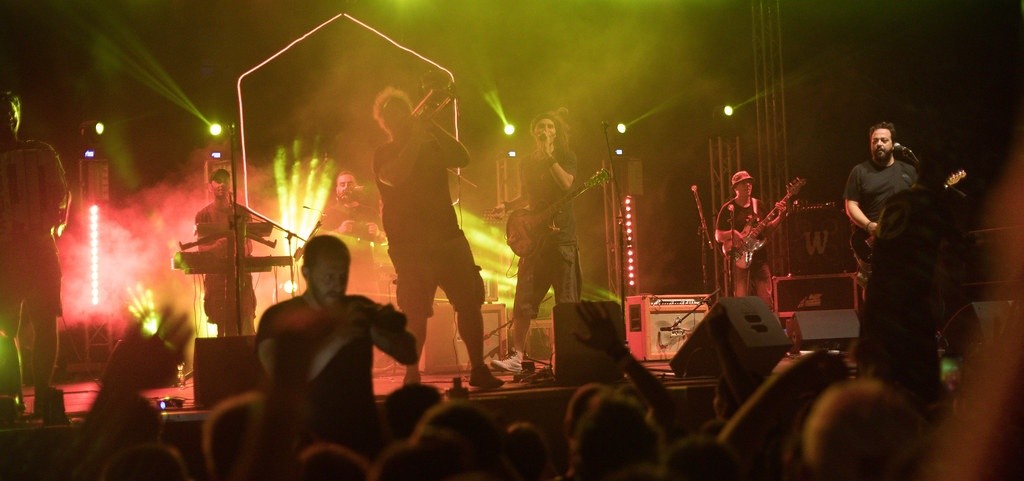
[176,361,184,385]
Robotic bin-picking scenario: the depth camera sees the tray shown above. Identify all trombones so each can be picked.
[405,70,458,130]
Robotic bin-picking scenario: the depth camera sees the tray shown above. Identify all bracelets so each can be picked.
[865,221,872,234]
[542,156,559,170]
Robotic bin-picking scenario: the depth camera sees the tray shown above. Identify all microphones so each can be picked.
[660,327,681,331]
[894,143,912,153]
[540,134,547,140]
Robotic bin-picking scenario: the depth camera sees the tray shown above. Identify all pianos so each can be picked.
[171,222,294,337]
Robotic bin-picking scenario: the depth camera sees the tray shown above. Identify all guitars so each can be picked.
[506,167,611,260]
[851,169,966,272]
[722,177,807,269]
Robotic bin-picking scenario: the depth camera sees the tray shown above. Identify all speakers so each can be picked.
[623,295,710,362]
[192,335,260,409]
[787,309,861,352]
[669,296,795,420]
[422,303,507,374]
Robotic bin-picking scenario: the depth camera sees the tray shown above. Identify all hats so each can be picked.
[731,170,753,191]
[210,169,230,183]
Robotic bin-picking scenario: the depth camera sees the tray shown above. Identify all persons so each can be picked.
[193,168,258,337]
[372,85,506,388]
[715,170,786,310]
[321,169,384,296]
[842,122,918,293]
[0,194,1024,481]
[0,89,72,415]
[489,109,581,376]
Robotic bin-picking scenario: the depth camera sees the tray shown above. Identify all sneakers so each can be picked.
[490,347,523,374]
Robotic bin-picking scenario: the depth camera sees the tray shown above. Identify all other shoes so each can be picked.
[468,365,504,389]
